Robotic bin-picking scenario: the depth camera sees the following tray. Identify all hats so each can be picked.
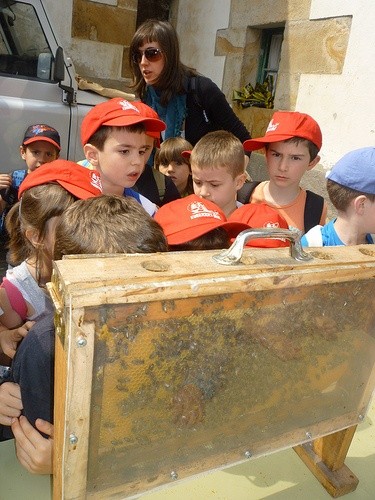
[326,147,375,196]
[225,202,295,249]
[21,124,62,152]
[18,160,105,202]
[152,194,255,247]
[79,98,167,145]
[181,150,193,166]
[243,109,322,155]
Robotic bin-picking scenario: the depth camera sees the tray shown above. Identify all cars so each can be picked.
[0,0,114,175]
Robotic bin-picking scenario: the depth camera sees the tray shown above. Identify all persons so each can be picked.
[1,97,375,476]
[126,20,258,181]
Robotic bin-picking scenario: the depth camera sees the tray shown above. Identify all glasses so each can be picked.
[129,46,171,63]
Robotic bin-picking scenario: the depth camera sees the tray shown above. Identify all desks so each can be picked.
[0,384,375,500]
